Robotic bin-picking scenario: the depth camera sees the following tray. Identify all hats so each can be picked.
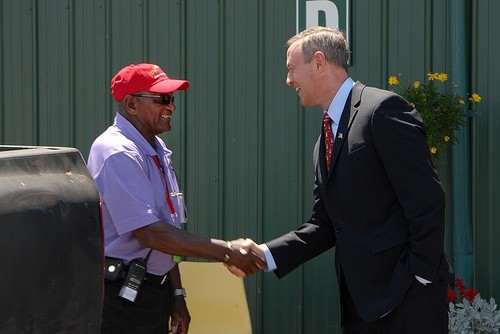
[111,63,190,102]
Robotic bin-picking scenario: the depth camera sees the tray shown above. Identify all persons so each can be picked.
[224,26,455,334]
[87,63,268,334]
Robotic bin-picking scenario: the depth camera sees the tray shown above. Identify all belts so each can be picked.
[143,272,170,285]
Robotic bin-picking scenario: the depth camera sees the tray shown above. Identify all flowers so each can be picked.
[386,71,487,169]
[448,279,500,334]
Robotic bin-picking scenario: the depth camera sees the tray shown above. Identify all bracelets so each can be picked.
[224,240,232,263]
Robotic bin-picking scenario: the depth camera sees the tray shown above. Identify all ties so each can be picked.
[323,112,334,172]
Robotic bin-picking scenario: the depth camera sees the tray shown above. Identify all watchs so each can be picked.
[173,288,186,297]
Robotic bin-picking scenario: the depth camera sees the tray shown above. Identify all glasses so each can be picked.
[130,94,176,106]
